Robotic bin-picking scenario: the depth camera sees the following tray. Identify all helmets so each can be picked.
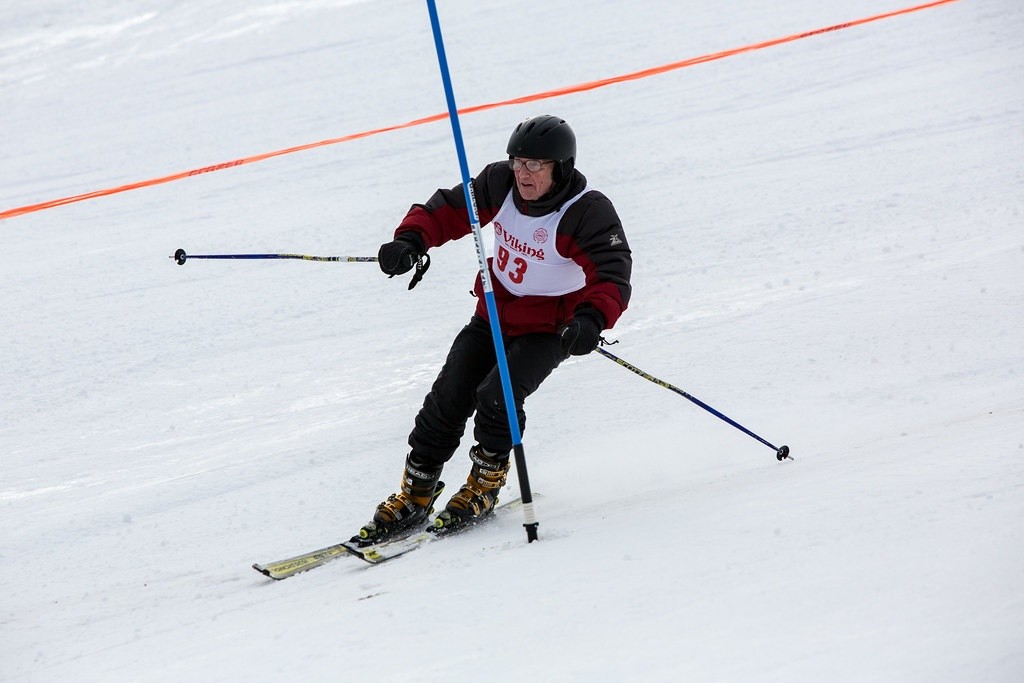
[506,115,576,181]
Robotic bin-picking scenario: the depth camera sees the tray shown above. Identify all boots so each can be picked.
[445,444,511,518]
[373,452,444,526]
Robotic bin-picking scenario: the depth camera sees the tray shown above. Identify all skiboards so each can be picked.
[251,491,548,581]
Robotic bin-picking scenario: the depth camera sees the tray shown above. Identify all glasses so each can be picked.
[508,159,554,172]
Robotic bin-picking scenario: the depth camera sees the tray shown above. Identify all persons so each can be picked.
[373,114,633,527]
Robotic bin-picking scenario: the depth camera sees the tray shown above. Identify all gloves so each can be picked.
[377,229,427,279]
[560,302,605,355]
